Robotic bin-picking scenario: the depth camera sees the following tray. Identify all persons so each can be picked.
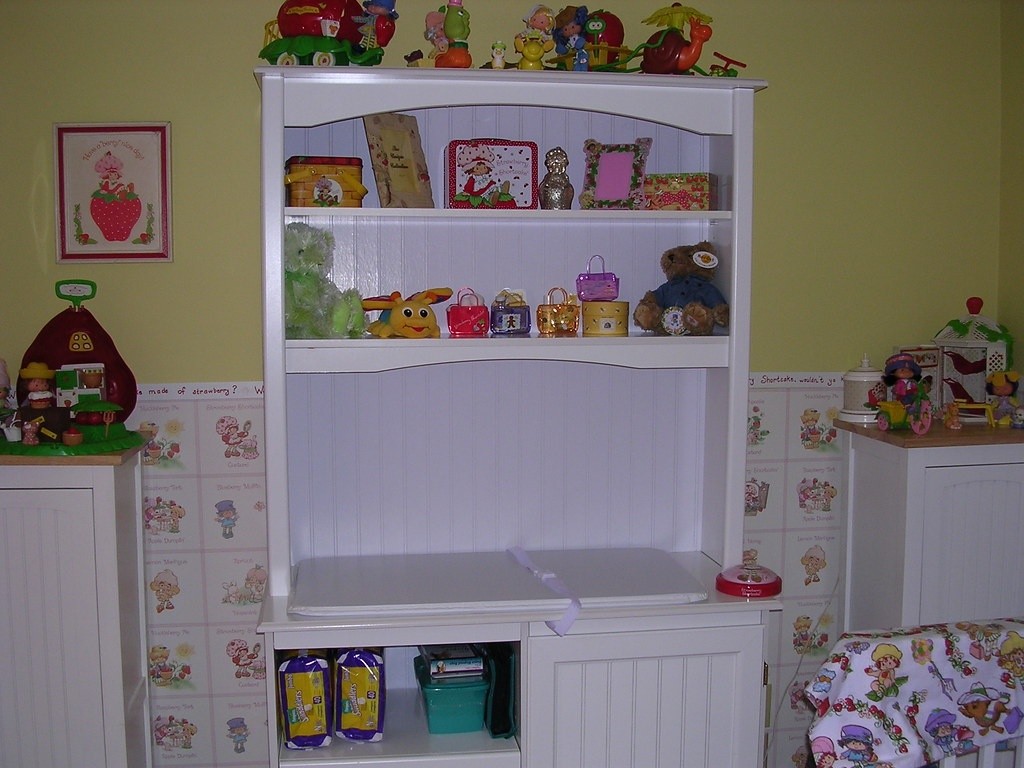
[518,5,588,74]
[986,371,1019,419]
[0,360,54,408]
[884,353,922,413]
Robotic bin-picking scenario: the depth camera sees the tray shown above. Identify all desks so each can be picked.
[0,430,156,767]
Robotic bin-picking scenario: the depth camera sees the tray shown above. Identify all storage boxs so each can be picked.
[414,658,492,735]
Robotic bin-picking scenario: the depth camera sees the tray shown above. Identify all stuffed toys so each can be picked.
[284,221,367,340]
[362,288,453,339]
[633,243,729,336]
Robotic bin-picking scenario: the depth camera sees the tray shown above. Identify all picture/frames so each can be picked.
[52,119,174,263]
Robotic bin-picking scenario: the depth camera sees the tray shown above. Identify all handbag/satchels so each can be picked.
[535,287,581,335]
[446,287,490,335]
[576,254,620,302]
[489,293,531,334]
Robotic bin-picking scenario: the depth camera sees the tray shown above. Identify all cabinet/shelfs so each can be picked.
[833,416,1024,628]
[251,66,775,768]
